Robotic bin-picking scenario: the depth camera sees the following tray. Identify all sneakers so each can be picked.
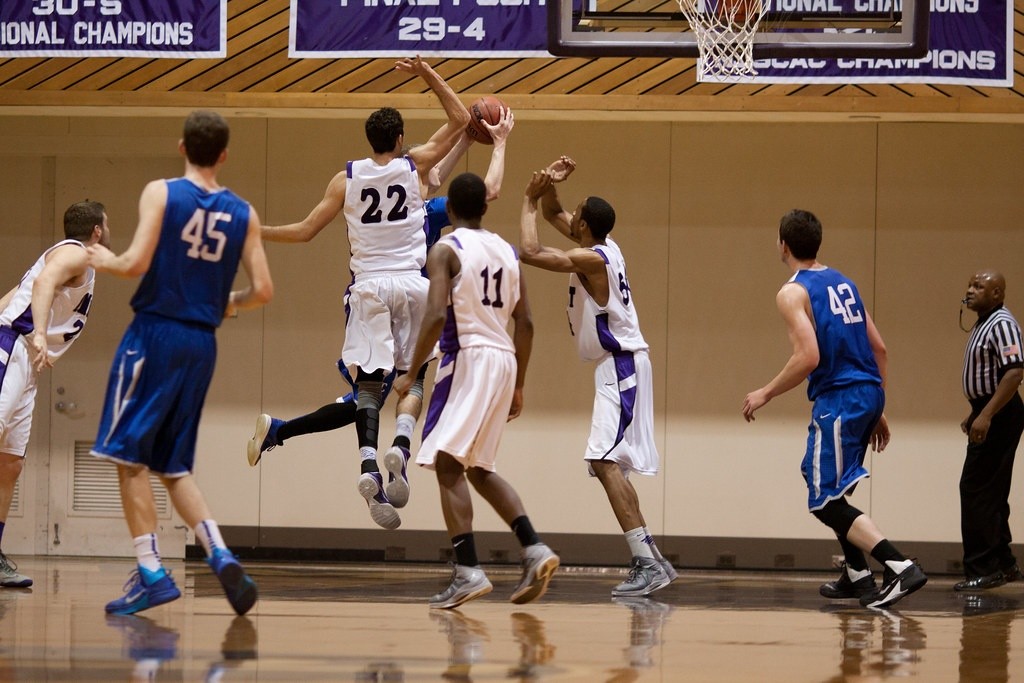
[819,559,877,600]
[0,554,33,587]
[222,615,258,666]
[656,555,680,582]
[430,562,493,608]
[612,555,669,597]
[953,563,1020,592]
[357,470,402,530]
[858,556,927,608]
[385,445,411,508]
[510,541,561,605]
[964,595,1020,615]
[104,613,179,662]
[105,569,180,615]
[247,413,286,466]
[204,549,257,616]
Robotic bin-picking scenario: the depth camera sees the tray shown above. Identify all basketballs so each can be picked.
[465,96,506,145]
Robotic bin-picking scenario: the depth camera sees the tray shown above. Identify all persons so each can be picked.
[394,173,561,609]
[953,266,1024,592]
[741,205,927,608]
[246,54,515,529]
[520,152,680,597]
[0,196,109,587]
[84,108,275,618]
